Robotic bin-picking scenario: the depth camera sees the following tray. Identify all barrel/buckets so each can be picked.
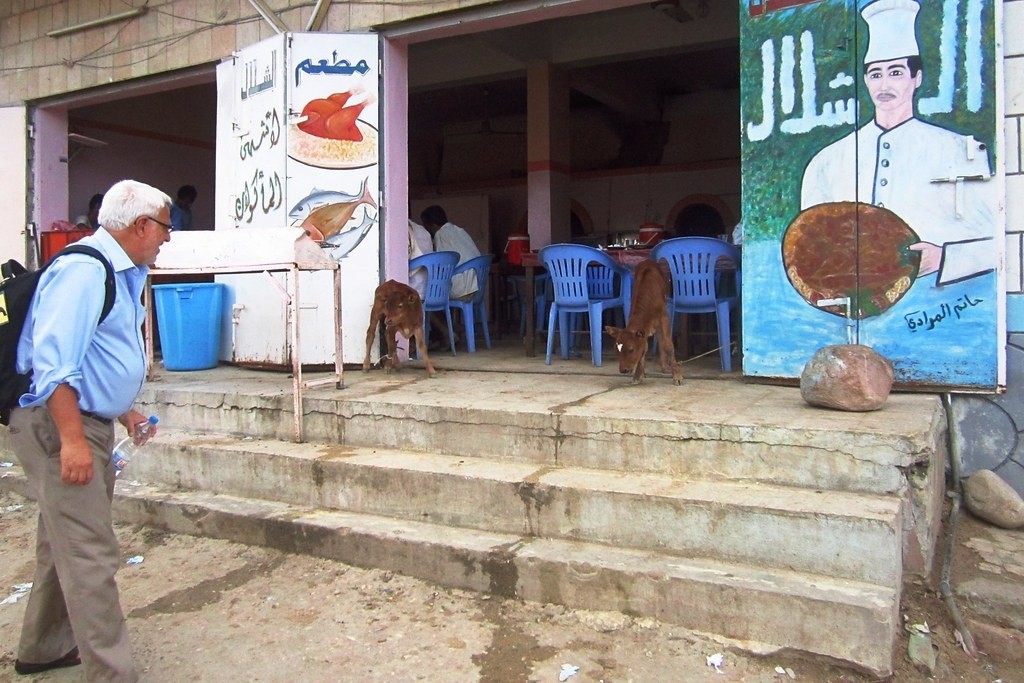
[150,284,227,371]
[639,223,664,248]
[504,233,530,265]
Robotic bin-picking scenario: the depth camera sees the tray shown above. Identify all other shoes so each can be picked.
[15,643,83,674]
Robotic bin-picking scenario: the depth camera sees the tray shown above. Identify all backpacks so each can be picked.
[1,245,117,427]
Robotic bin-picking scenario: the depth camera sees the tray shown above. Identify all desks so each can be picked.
[521,245,741,361]
[490,264,526,341]
[141,226,341,443]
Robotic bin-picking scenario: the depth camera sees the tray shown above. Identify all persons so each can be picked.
[13,179,174,683]
[692,216,742,357]
[420,204,482,351]
[76,194,105,229]
[408,200,434,357]
[169,185,197,231]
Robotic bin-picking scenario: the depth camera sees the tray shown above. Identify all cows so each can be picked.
[603,260,684,387]
[361,279,438,379]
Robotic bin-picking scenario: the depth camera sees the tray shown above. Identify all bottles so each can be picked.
[113,415,159,478]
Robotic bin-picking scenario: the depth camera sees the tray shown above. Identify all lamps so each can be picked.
[45,8,145,41]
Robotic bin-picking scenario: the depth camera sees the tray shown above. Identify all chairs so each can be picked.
[409,251,455,354]
[447,254,495,353]
[510,272,550,338]
[580,265,623,327]
[538,244,634,368]
[651,237,742,372]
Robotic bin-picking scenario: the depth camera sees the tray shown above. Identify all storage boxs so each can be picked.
[40,230,93,264]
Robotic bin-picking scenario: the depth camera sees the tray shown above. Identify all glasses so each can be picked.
[133,215,176,234]
